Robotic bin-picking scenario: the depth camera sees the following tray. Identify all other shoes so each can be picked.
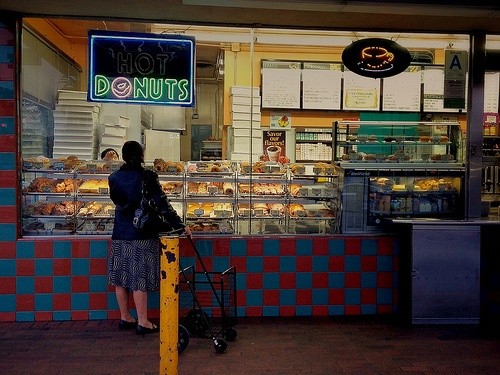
[136,322,159,335]
[119,318,138,330]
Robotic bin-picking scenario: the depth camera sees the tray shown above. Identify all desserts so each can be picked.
[342,136,449,160]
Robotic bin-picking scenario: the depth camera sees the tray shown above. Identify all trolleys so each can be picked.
[154,225,239,355]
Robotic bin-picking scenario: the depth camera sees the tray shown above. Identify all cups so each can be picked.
[266,146,281,162]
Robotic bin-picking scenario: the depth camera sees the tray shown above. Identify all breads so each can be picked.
[238,161,337,219]
[21,155,184,218]
[186,162,233,217]
[420,179,457,190]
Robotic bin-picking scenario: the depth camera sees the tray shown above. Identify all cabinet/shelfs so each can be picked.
[331,119,461,165]
[20,156,340,235]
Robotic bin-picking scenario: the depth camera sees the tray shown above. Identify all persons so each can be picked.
[108,141,193,334]
[101,149,119,160]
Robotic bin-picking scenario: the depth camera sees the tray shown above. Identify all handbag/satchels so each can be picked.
[132,197,173,234]
